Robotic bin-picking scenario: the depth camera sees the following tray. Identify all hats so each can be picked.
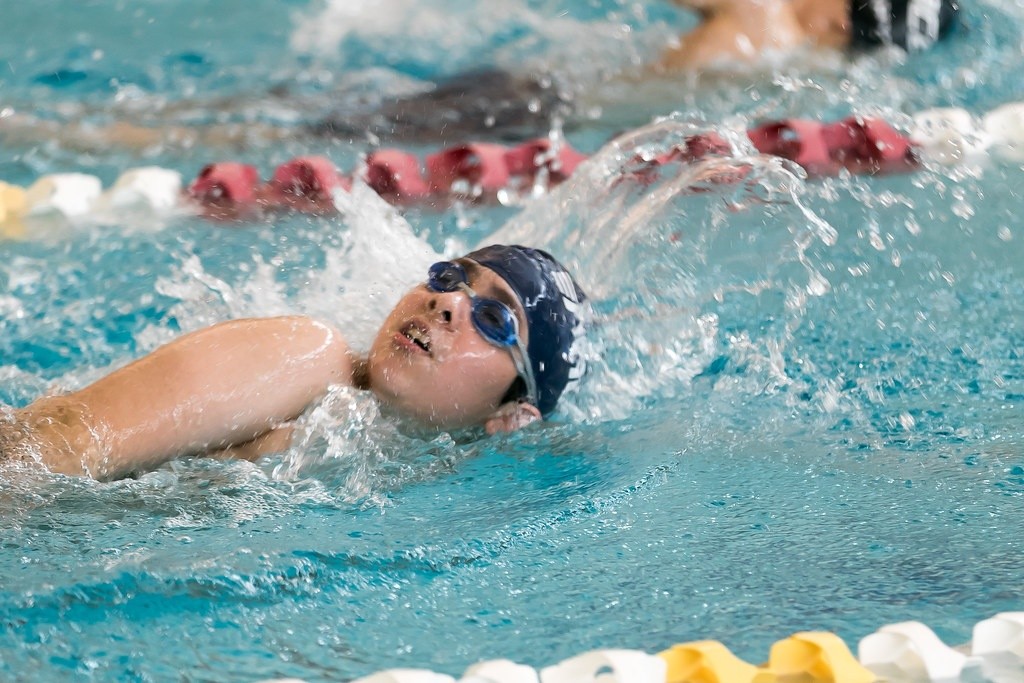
[460,243,595,423]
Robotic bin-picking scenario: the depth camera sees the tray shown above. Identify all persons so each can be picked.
[1,0,957,162]
[0,244,588,525]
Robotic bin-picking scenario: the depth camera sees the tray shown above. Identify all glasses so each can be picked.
[425,260,539,406]
